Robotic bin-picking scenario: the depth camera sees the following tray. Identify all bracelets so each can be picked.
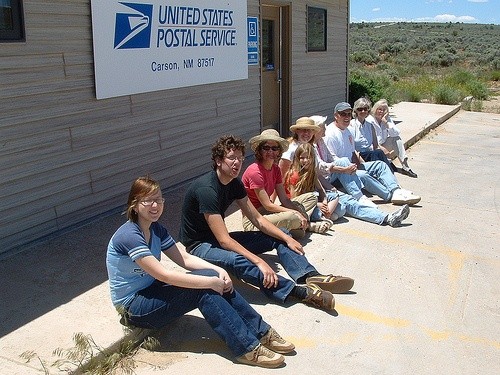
[385,120,388,124]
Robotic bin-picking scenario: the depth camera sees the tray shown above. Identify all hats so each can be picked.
[309,115,327,125]
[334,102,353,115]
[249,129,290,153]
[289,117,320,133]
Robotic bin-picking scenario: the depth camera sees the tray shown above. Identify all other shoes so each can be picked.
[320,218,333,227]
[391,189,421,205]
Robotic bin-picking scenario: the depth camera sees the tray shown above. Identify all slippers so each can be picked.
[290,229,304,239]
[309,221,330,234]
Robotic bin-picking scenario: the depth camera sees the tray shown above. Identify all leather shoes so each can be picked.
[401,168,417,178]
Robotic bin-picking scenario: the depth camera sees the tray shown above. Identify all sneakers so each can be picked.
[306,274,354,294]
[302,282,335,310]
[259,327,295,354]
[387,204,410,227]
[233,342,285,368]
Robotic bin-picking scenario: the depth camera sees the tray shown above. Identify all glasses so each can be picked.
[338,112,352,116]
[135,198,165,206]
[357,108,368,111]
[261,145,280,151]
[222,155,245,161]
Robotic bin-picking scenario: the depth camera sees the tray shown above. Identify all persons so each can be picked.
[270,118,410,227]
[240,129,318,239]
[347,97,394,174]
[281,143,346,227]
[324,102,421,205]
[178,135,354,310]
[365,99,417,178]
[309,114,376,205]
[106,177,295,367]
